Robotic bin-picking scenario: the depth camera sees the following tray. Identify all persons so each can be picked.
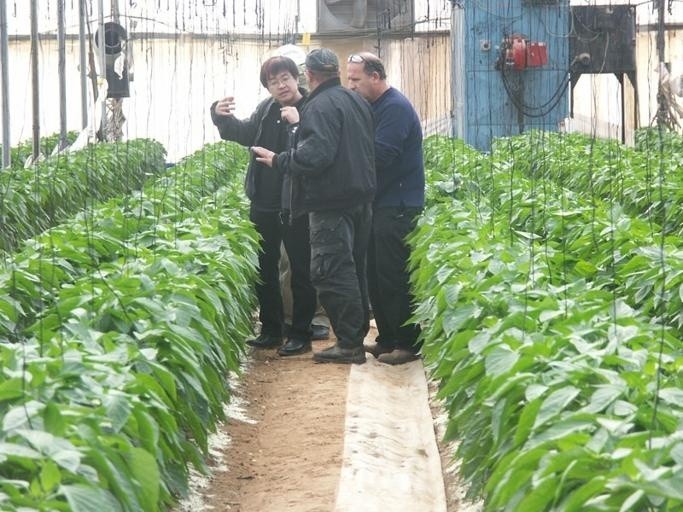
[276,235,331,341]
[344,50,425,365]
[209,55,317,357]
[250,48,378,365]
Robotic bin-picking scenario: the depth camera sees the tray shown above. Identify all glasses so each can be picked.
[346,52,368,64]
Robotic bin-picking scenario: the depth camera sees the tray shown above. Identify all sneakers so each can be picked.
[366,341,419,364]
[313,346,366,363]
[310,323,329,340]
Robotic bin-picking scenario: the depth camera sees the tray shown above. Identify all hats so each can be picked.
[304,47,340,75]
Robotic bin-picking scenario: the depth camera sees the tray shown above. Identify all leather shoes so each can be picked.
[246,333,313,355]
